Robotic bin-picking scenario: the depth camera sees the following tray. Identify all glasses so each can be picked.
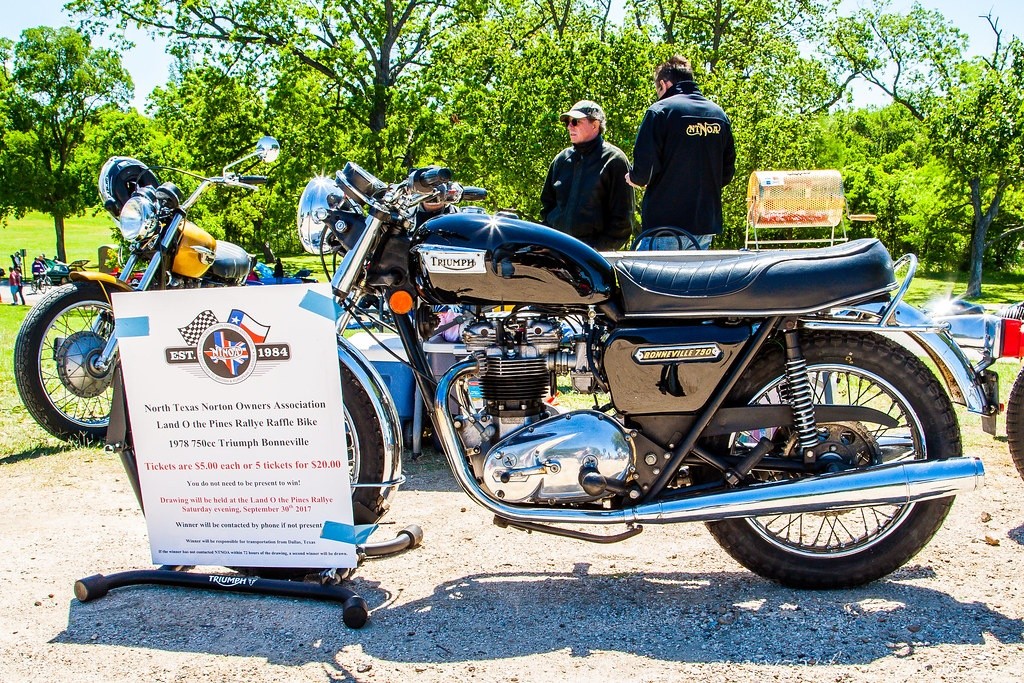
[565,118,579,127]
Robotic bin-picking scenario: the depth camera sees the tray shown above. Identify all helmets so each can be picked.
[34,257,39,260]
[98,156,161,220]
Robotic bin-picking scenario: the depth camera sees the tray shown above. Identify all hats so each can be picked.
[560,100,606,130]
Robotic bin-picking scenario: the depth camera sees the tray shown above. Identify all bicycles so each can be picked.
[30,268,52,294]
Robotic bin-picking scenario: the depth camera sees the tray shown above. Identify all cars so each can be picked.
[41,257,90,287]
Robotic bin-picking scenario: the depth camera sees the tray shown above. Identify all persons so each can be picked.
[625,54,735,251]
[540,99,635,252]
[9,252,50,304]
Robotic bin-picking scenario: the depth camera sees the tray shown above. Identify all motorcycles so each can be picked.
[10,135,1024,593]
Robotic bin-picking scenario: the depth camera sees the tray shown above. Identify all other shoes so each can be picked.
[11,302,18,305]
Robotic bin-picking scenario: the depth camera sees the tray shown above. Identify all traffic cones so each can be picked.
[0,293,4,304]
